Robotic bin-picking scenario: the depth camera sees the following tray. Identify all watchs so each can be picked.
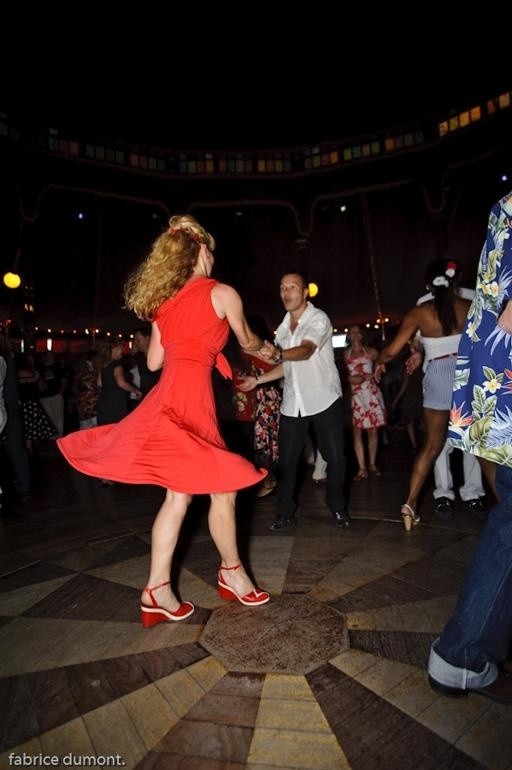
[274,352,282,362]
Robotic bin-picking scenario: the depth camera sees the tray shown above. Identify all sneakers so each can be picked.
[464,499,484,512]
[435,500,454,516]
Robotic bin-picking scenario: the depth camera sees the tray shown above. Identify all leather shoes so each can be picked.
[334,511,351,528]
[269,515,297,531]
[427,668,512,705]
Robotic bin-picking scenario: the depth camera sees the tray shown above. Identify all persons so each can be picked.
[133,327,164,400]
[427,192,512,704]
[376,256,501,534]
[57,215,266,627]
[235,273,352,533]
[341,322,386,482]
[38,349,67,441]
[15,354,59,455]
[68,361,99,430]
[0,355,8,435]
[0,324,33,495]
[87,347,104,388]
[95,339,143,488]
[305,447,328,487]
[406,254,490,520]
[391,344,426,450]
[251,358,282,498]
[224,362,257,468]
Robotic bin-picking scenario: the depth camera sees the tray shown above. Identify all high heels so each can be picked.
[139,580,195,629]
[401,503,422,530]
[216,565,270,606]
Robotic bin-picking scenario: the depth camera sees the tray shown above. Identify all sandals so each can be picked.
[370,470,383,480]
[356,469,369,481]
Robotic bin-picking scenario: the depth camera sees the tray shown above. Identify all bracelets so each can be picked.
[255,376,260,387]
